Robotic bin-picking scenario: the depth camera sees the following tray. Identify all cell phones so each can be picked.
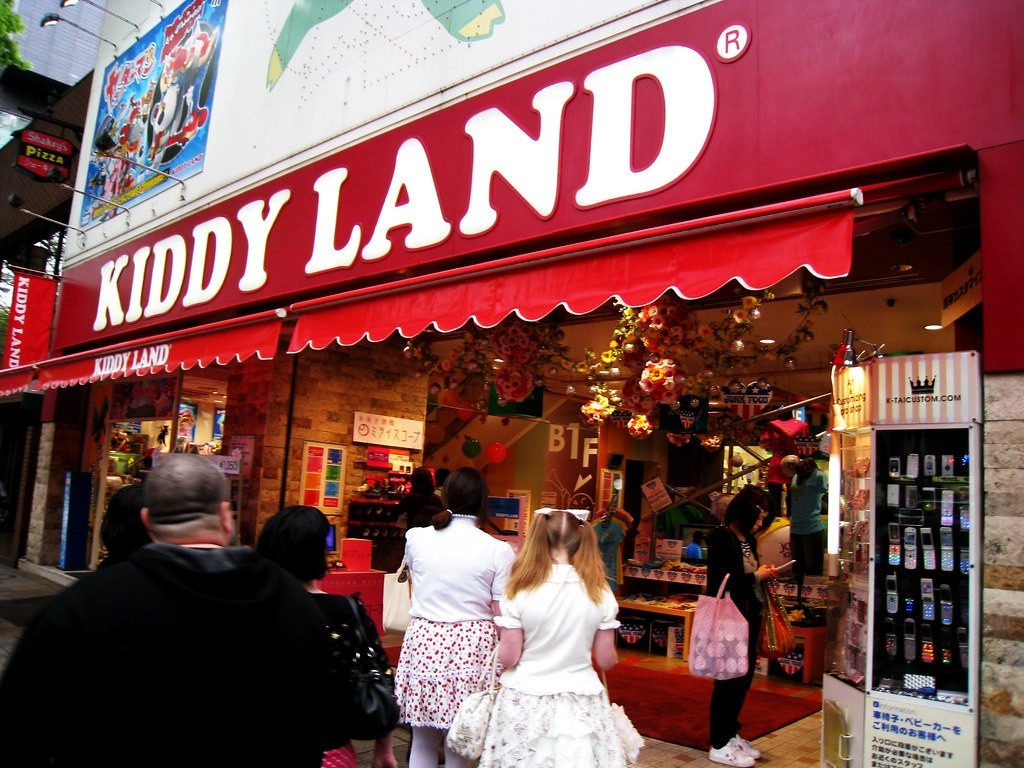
[773,560,795,572]
[880,453,969,691]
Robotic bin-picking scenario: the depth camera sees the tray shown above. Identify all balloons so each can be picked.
[462,438,508,465]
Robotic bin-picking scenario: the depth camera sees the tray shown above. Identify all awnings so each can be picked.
[0,162,980,404]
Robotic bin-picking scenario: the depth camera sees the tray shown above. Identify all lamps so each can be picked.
[40,0,143,59]
[1,129,188,279]
[830,328,882,367]
[830,365,848,432]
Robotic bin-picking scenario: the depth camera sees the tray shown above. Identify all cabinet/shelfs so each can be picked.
[348,496,411,530]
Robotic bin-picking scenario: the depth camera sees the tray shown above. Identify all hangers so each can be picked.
[587,495,633,535]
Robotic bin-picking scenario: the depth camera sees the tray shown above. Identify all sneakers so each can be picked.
[709,743,757,768]
[728,732,762,762]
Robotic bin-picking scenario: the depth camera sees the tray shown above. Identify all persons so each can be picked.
[762,405,799,519]
[0,453,399,768]
[478,507,644,768]
[782,457,827,587]
[686,530,704,559]
[400,467,453,529]
[394,466,516,768]
[97,484,398,768]
[704,485,777,767]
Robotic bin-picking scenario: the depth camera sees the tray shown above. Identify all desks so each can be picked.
[761,622,827,683]
[612,595,697,661]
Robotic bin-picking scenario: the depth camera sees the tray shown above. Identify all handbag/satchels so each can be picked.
[688,573,749,681]
[760,573,795,659]
[337,593,399,741]
[446,642,501,760]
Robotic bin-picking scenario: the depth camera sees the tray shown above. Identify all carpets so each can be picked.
[382,645,824,753]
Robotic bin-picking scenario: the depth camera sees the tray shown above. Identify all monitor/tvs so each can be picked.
[325,521,341,556]
[608,454,623,470]
